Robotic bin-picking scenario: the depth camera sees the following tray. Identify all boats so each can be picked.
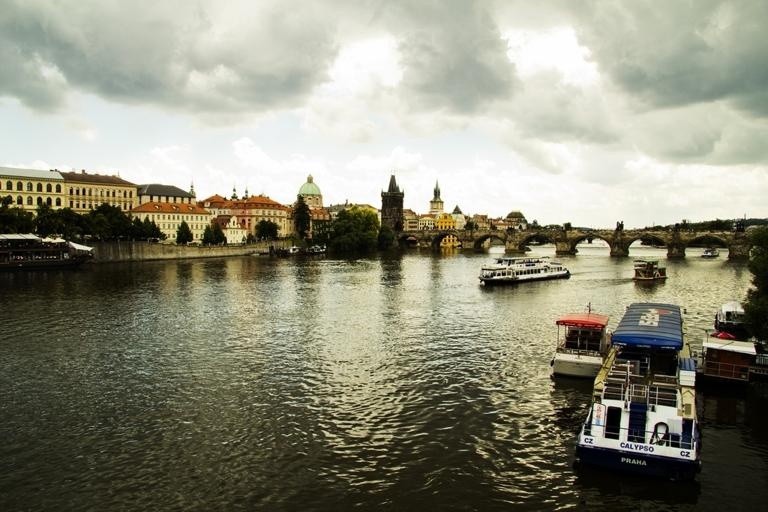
[479,255,568,285]
[304,243,326,256]
[714,300,752,332]
[551,302,612,380]
[701,244,720,258]
[575,302,701,491]
[0,233,94,272]
[279,244,306,257]
[631,259,668,281]
